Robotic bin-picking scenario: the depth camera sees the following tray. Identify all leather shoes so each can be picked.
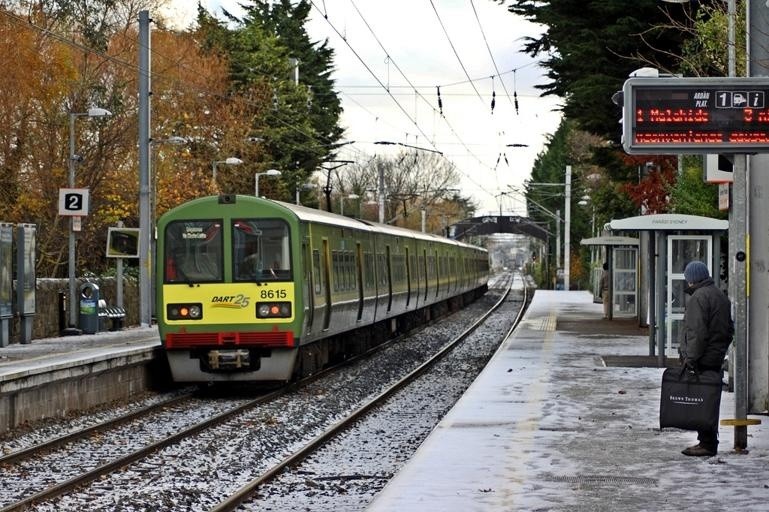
[681,442,717,456]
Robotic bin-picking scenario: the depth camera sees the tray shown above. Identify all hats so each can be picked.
[684,260,709,283]
[603,262,608,270]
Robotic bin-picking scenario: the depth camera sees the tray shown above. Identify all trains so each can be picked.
[153,193,491,388]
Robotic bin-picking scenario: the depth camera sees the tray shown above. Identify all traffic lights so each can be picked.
[110,230,135,255]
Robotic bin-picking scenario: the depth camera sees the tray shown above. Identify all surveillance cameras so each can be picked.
[73,154,83,162]
[612,91,624,106]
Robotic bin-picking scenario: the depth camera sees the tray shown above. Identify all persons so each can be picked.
[599,262,609,319]
[680,260,733,456]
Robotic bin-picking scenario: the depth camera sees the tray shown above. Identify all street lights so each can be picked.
[501,165,573,290]
[67,107,111,331]
[255,168,282,197]
[148,136,188,326]
[296,183,318,205]
[211,158,243,196]
[340,194,360,216]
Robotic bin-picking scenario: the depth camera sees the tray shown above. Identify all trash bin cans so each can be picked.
[78,282,100,335]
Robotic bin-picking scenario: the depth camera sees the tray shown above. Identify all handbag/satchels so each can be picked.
[660,362,721,434]
